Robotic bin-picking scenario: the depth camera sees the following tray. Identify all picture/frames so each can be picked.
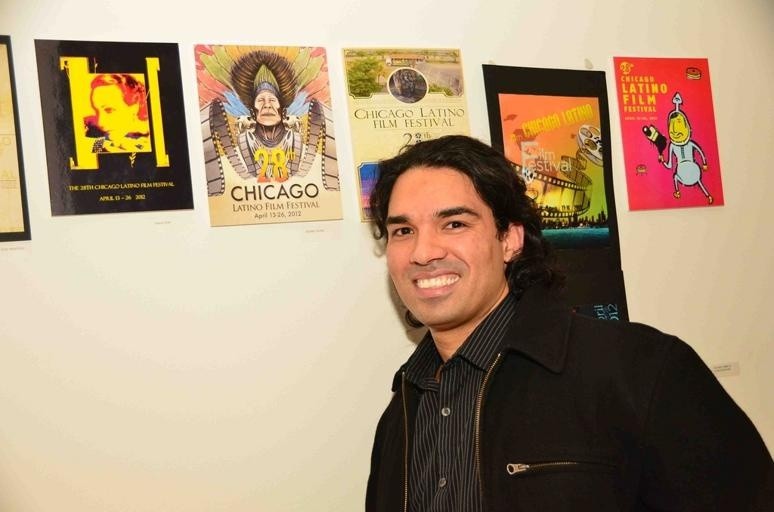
[0,35,31,242]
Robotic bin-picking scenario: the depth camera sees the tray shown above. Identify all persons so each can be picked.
[363,134,773,511]
[228,51,308,180]
[90,70,154,153]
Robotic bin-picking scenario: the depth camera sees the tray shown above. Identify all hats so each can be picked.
[250,64,282,105]
